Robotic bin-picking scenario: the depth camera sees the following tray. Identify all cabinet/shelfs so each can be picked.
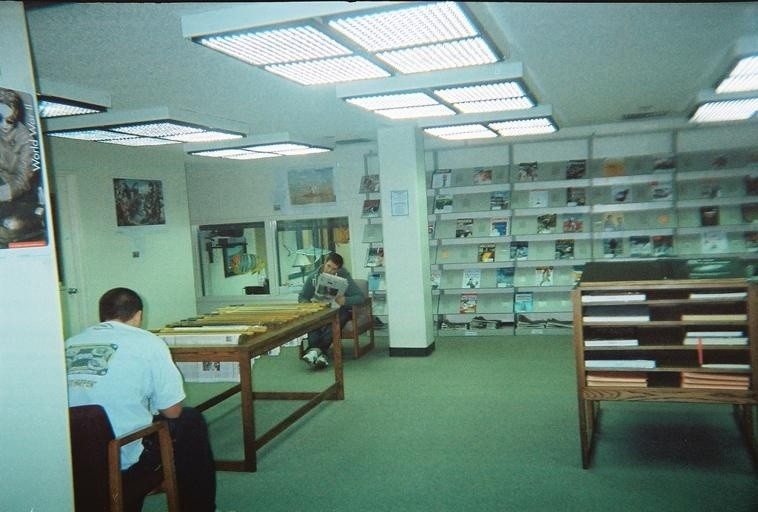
[571,258,758,475]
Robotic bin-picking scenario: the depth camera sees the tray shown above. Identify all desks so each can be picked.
[155,304,345,473]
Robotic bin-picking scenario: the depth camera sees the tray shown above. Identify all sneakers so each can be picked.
[303,351,328,368]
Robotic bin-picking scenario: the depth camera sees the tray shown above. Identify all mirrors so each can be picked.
[198,222,270,295]
[276,216,352,289]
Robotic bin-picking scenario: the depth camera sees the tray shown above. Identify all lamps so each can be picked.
[291,254,312,285]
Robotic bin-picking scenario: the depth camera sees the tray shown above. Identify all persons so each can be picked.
[298,250,365,369]
[0,87,44,249]
[58,287,217,511]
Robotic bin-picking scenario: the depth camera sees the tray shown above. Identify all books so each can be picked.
[598,154,679,257]
[427,168,510,239]
[517,158,587,234]
[698,152,757,252]
[359,175,388,329]
[429,240,533,314]
[534,239,585,287]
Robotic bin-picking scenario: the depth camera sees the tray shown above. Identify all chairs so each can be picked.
[297,280,376,359]
[70,404,180,512]
